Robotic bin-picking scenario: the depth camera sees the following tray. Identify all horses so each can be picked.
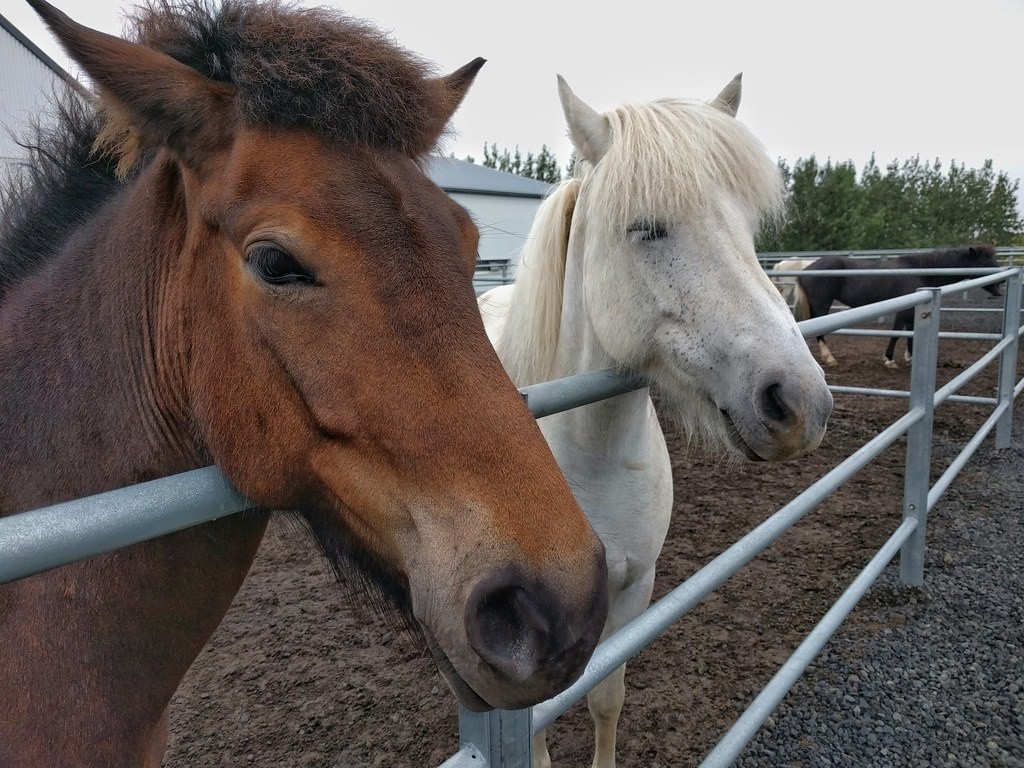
[471,69,833,768]
[770,243,1008,370]
[0,0,610,768]
